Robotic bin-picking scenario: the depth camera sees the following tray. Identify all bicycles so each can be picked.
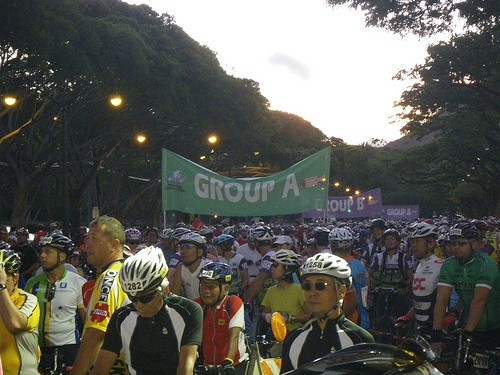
[32,242,500,375]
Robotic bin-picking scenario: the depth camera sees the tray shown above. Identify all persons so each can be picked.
[0,214,500,375]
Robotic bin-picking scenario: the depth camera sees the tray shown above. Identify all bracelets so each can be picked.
[291,315,295,324]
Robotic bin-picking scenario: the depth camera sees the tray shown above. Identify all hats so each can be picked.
[272,236,292,247]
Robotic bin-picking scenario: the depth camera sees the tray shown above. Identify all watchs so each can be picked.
[0,284,7,291]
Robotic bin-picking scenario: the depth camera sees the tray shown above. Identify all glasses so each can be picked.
[48,284,56,302]
[302,280,332,291]
[452,242,466,246]
[180,243,191,249]
[127,291,157,303]
[129,241,140,245]
[271,264,279,268]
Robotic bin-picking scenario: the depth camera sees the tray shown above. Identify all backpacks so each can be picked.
[226,295,256,343]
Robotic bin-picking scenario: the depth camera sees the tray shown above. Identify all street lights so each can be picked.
[63,93,122,227]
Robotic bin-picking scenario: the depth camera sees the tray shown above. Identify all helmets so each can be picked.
[16,227,30,235]
[125,228,141,240]
[119,246,169,296]
[160,222,216,240]
[215,235,234,245]
[0,249,22,273]
[222,217,276,240]
[39,234,74,252]
[406,221,438,239]
[438,216,500,242]
[284,225,371,247]
[371,218,403,240]
[196,262,233,286]
[269,249,303,265]
[300,253,352,278]
[177,232,208,246]
